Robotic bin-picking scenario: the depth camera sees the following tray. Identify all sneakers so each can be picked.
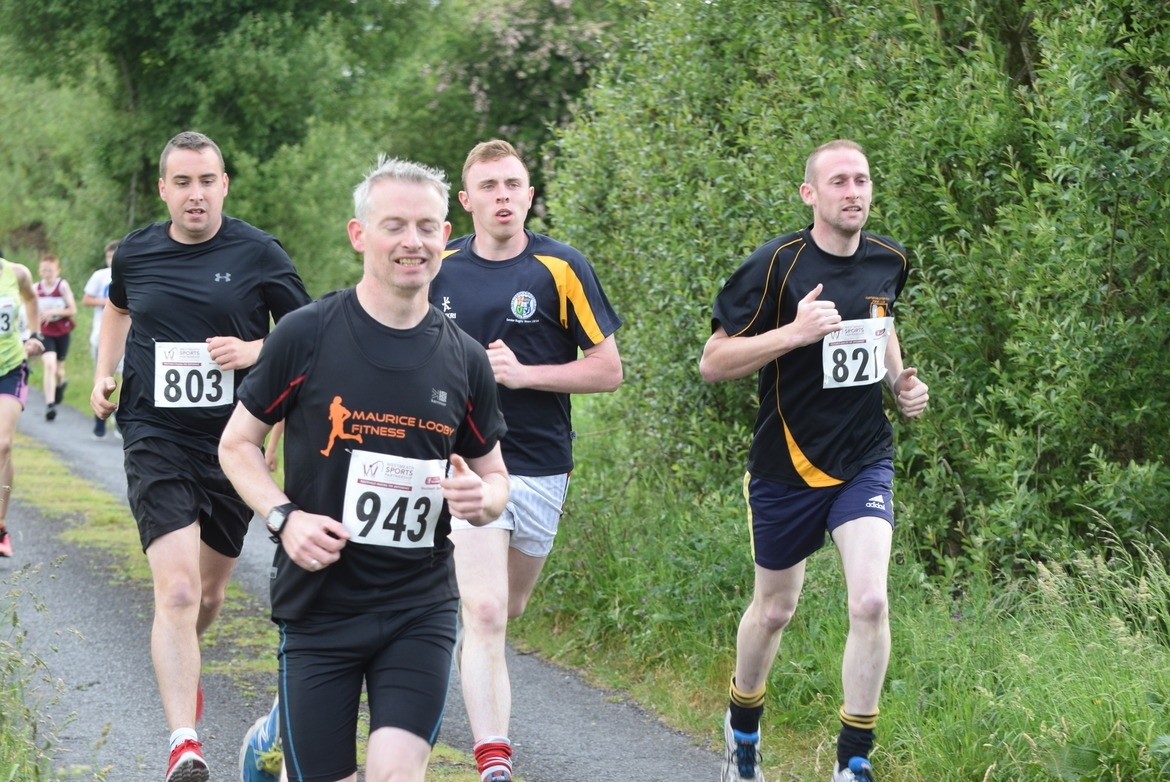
[92,414,108,441]
[195,683,204,724]
[238,691,287,782]
[720,706,764,782]
[0,525,13,558]
[481,766,513,782]
[165,739,210,782]
[112,411,123,439]
[831,756,875,782]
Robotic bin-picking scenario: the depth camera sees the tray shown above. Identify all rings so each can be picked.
[310,560,317,570]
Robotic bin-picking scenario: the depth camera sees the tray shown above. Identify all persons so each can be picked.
[33,253,77,421]
[90,131,313,782]
[0,256,45,557]
[83,240,123,440]
[427,140,624,782]
[699,140,930,782]
[217,158,510,782]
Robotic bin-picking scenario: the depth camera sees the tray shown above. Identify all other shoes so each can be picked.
[56,380,68,404]
[46,403,56,421]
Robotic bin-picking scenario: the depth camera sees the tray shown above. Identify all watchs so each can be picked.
[30,332,45,343]
[265,503,299,544]
